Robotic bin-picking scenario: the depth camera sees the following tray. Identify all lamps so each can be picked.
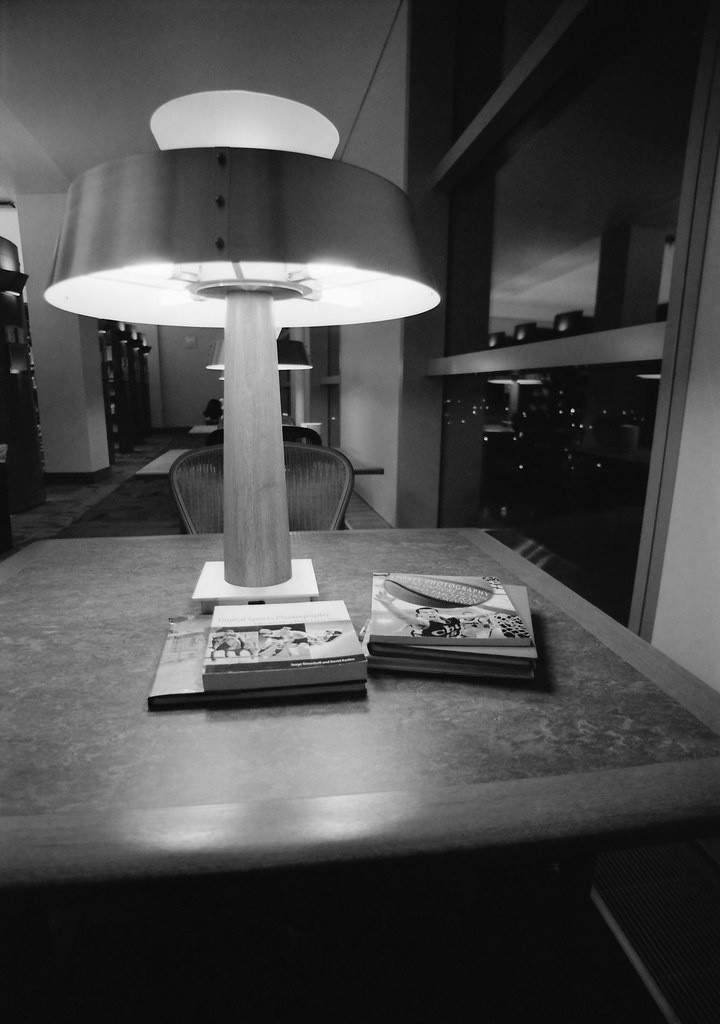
[133,340,143,350]
[204,334,314,430]
[119,331,132,343]
[143,346,152,355]
[41,87,443,620]
[3,272,29,297]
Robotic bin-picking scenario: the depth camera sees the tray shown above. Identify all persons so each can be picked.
[371,589,487,638]
[211,623,341,661]
[457,610,483,638]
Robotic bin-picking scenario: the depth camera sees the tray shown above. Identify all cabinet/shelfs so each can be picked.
[0,234,152,553]
[393,0,720,656]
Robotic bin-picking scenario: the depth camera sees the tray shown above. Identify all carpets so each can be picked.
[590,840,720,1024]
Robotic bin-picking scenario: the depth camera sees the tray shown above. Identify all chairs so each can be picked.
[167,443,361,532]
[207,426,321,447]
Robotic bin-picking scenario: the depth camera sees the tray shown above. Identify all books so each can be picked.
[147,614,367,707]
[202,599,367,691]
[359,572,539,678]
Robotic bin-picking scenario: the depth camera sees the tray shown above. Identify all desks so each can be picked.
[0,526,720,947]
[189,424,217,434]
[135,449,386,475]
